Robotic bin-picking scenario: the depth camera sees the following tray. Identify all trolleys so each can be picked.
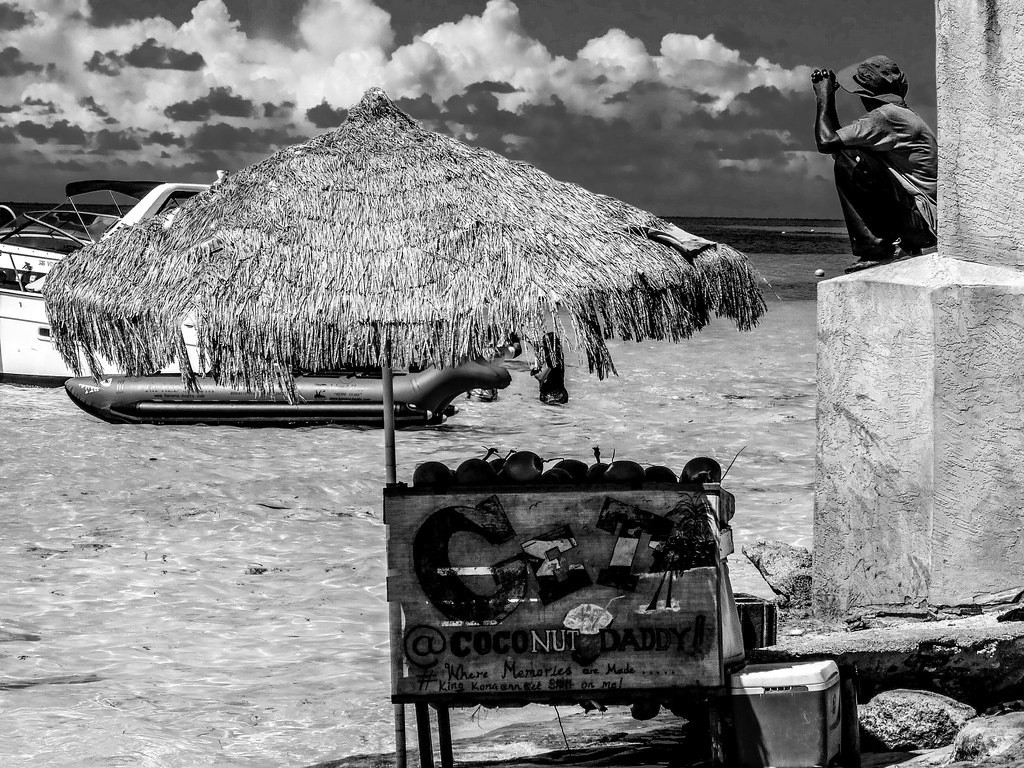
[382,474,751,768]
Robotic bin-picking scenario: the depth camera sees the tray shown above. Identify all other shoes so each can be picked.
[845,244,923,274]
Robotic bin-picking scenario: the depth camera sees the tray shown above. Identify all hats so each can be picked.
[837,55,909,106]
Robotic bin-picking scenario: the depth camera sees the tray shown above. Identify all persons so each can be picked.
[812,55,944,275]
[466,325,569,405]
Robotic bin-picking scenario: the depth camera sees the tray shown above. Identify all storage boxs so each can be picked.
[732,660,841,767]
[734,592,780,648]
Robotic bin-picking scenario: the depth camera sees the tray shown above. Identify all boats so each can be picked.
[64,351,513,426]
[0,168,231,380]
